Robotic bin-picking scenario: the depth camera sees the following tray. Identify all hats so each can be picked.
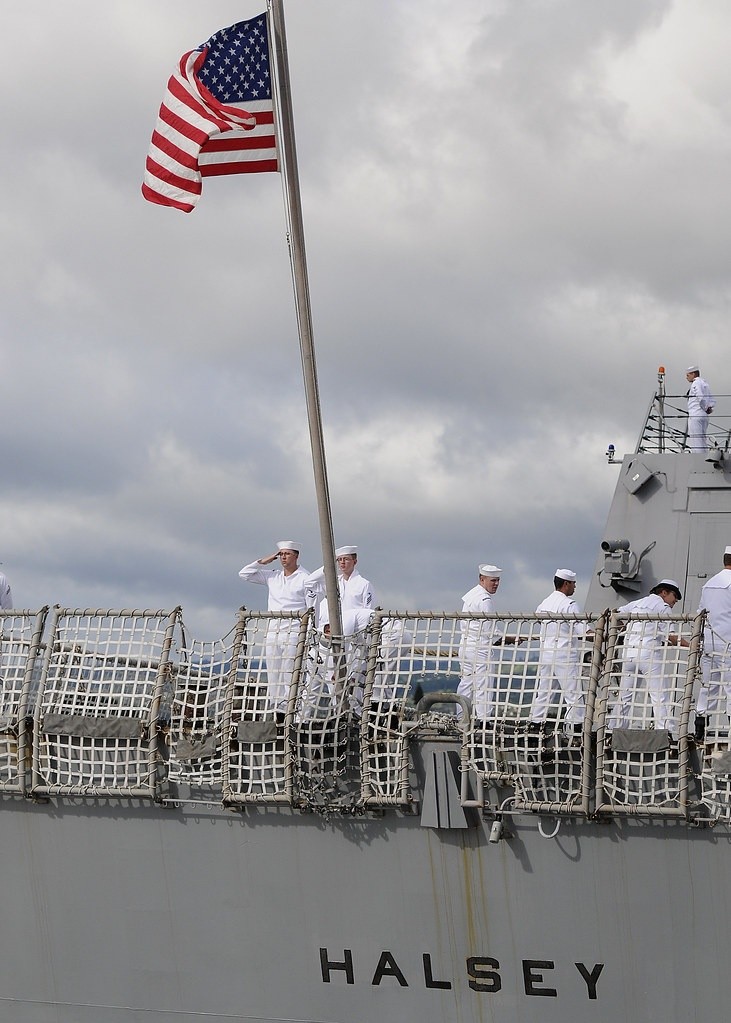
[686,366,698,373]
[725,545,731,554]
[660,579,679,589]
[276,541,302,552]
[479,564,503,577]
[555,569,577,581]
[335,546,358,556]
[316,623,331,649]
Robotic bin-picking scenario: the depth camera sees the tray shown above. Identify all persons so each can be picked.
[694,545,731,744]
[304,548,373,716]
[531,569,609,734]
[605,579,698,743]
[238,541,318,727]
[320,607,413,712]
[456,563,523,730]
[685,366,715,453]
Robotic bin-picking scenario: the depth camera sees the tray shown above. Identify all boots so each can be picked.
[370,702,384,725]
[694,717,709,739]
[384,704,398,731]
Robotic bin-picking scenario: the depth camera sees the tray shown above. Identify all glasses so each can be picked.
[279,552,297,557]
[337,557,356,562]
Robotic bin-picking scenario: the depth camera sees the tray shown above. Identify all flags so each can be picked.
[141,15,280,215]
